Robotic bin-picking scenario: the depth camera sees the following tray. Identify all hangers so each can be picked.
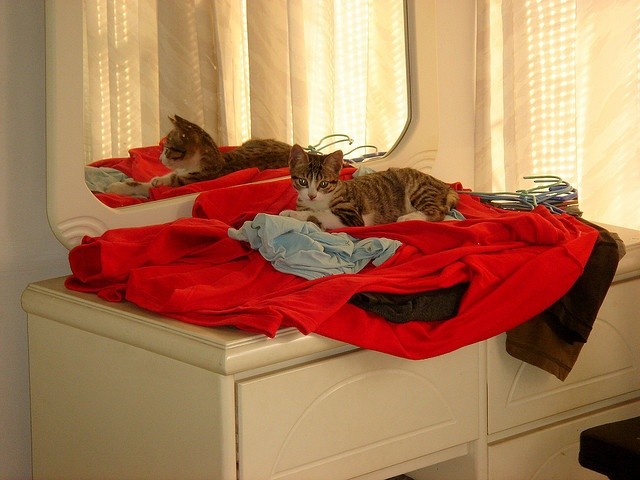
[456,175,579,230]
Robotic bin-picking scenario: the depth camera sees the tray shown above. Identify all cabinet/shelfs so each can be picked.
[223,275,640,480]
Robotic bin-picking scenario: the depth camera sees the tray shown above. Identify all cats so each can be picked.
[278,143,460,231]
[149,113,292,189]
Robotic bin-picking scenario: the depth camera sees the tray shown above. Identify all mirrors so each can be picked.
[44,2,439,248]
